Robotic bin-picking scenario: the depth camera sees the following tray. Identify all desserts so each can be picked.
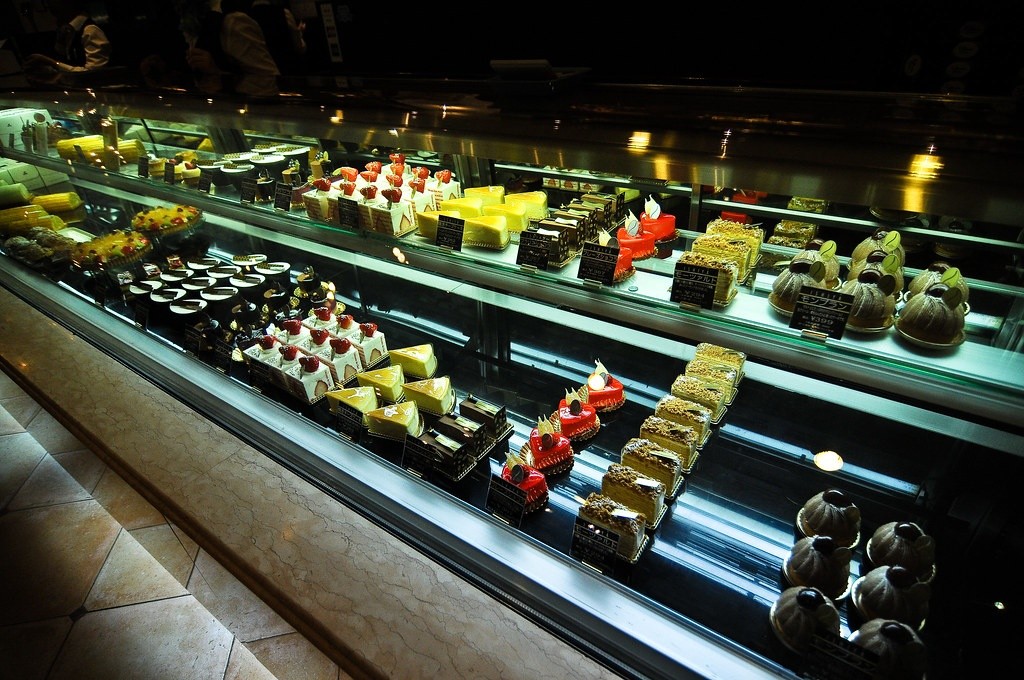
[0,108,969,680]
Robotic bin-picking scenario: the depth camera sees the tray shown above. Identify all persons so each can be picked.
[141,0,279,100]
[20,0,113,90]
[250,0,302,92]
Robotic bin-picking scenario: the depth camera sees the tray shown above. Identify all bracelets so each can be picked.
[53,62,60,68]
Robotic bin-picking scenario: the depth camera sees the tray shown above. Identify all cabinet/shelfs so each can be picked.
[0,91,1024,680]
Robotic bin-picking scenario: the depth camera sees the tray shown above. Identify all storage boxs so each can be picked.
[0,169,14,185]
[35,155,69,187]
[0,114,25,147]
[7,162,45,191]
[17,109,54,126]
